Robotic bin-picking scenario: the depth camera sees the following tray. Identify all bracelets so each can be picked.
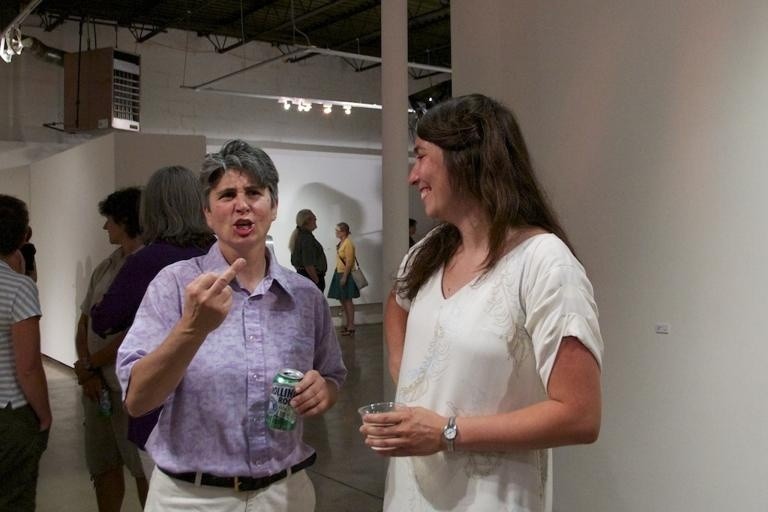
[84,360,94,374]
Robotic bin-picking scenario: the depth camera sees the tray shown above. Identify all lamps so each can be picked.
[178,0,419,123]
[0,0,45,64]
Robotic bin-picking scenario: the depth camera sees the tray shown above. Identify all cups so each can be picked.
[356,401,406,456]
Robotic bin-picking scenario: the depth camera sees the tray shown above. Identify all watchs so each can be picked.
[443,417,457,452]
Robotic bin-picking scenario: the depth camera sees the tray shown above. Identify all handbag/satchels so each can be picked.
[351,269,368,290]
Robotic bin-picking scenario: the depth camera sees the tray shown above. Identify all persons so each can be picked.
[4,249,25,276]
[409,217,418,248]
[115,136,349,511]
[358,90,603,512]
[328,220,361,336]
[71,185,149,512]
[286,209,328,295]
[0,196,53,512]
[20,225,37,284]
[88,163,219,452]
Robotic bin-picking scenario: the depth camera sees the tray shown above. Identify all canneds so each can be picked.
[265,367,305,435]
[95,385,113,417]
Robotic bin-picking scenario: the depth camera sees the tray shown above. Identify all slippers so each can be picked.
[341,328,355,336]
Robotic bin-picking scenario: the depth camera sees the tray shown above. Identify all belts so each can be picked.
[156,452,318,490]
